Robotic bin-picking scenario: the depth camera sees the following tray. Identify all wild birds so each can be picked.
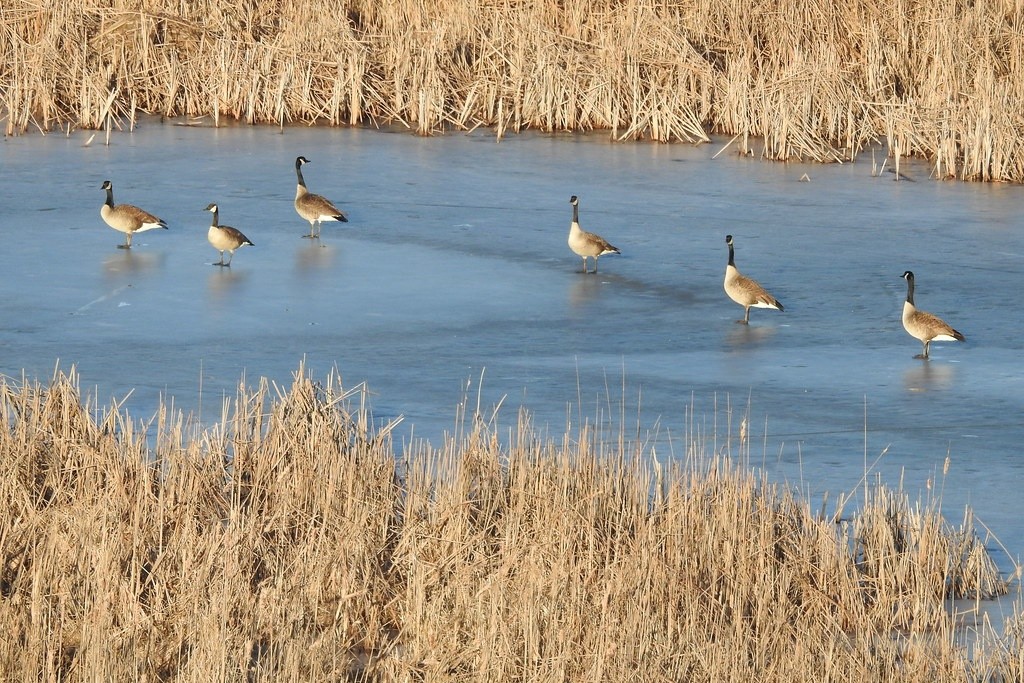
[567,196,621,274]
[724,235,785,325]
[99,180,168,249]
[899,270,965,359]
[202,203,256,268]
[294,156,349,239]
